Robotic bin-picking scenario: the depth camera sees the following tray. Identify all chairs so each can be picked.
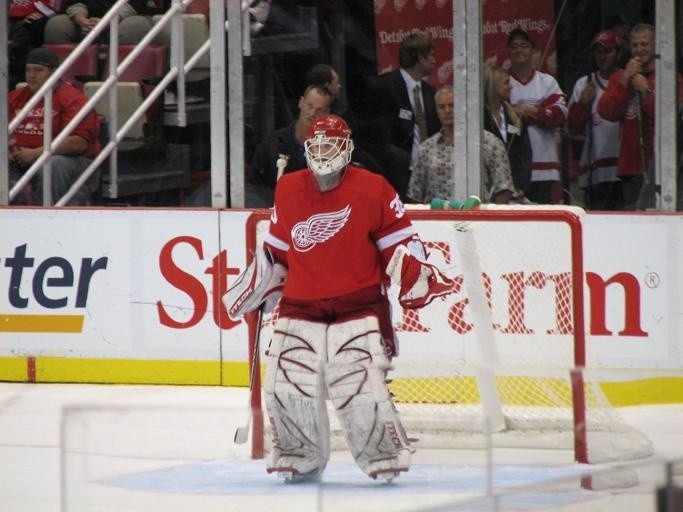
[9,0,209,207]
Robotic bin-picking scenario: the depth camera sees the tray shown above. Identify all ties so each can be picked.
[412,85,428,142]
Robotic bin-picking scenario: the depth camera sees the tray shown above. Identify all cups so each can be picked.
[9,138,21,154]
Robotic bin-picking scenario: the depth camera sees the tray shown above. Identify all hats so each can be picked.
[591,31,620,48]
[26,47,59,68]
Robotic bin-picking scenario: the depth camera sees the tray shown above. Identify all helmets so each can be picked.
[303,114,354,193]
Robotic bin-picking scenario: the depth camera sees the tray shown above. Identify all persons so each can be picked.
[558,1,592,61]
[567,27,623,211]
[505,27,569,204]
[406,84,515,204]
[222,114,456,485]
[603,10,632,43]
[44,0,171,47]
[248,86,333,206]
[319,2,369,66]
[348,33,442,204]
[596,23,656,211]
[484,65,534,204]
[6,48,101,210]
[304,65,342,99]
[6,0,61,91]
[191,123,276,209]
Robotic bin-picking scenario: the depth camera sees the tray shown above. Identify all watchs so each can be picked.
[641,89,653,95]
